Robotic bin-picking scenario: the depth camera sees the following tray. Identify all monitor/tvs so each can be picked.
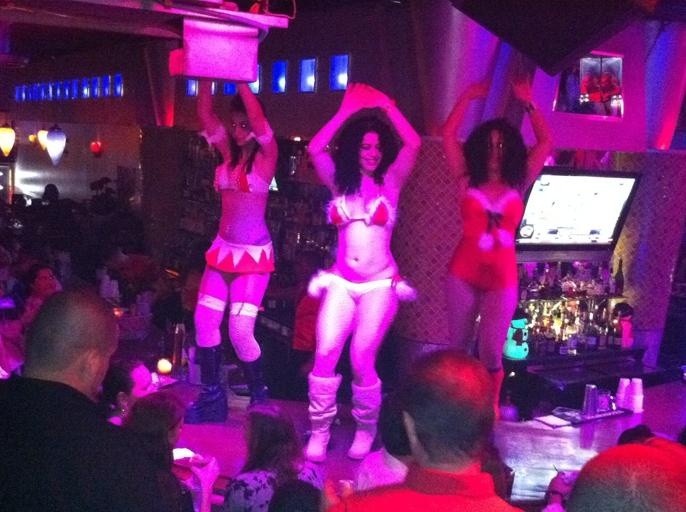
[515,166,642,251]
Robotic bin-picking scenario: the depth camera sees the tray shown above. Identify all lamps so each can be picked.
[0,107,106,167]
[187,53,350,97]
[11,73,123,101]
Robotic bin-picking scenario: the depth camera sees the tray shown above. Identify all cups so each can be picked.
[581,377,645,416]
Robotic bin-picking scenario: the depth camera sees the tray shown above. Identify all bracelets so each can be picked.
[381,99,397,112]
[525,100,538,112]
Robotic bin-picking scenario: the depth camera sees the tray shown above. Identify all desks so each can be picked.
[502,317,646,423]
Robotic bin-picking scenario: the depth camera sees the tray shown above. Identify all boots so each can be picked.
[243,352,270,404]
[306,371,342,462]
[184,344,227,424]
[348,377,382,459]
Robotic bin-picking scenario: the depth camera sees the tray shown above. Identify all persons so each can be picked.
[302,82,423,464]
[544,425,685,512]
[1,185,323,512]
[184,78,280,425]
[442,74,557,429]
[325,351,521,510]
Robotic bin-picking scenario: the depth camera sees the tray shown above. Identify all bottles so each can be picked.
[524,257,622,355]
